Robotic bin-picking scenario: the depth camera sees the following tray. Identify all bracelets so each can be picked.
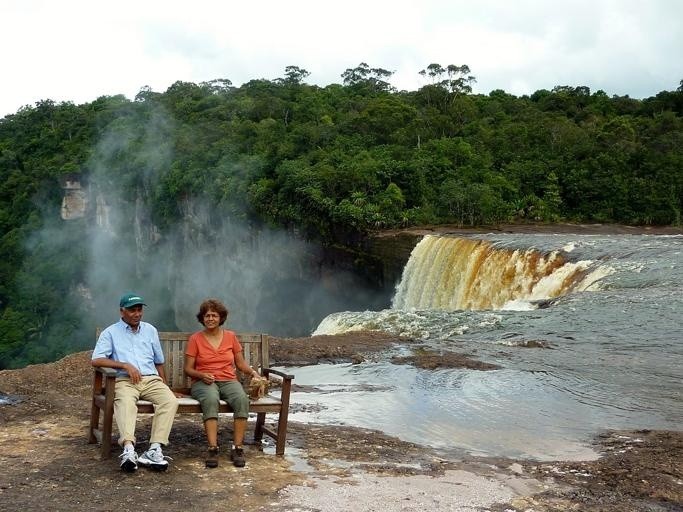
[248,371,256,376]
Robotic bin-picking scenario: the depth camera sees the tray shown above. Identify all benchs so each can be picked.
[89,326,295,460]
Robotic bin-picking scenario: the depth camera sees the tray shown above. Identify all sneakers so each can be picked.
[230,443,245,467]
[204,445,218,468]
[137,447,168,471]
[118,446,138,472]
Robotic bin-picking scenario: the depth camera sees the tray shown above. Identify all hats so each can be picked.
[120,293,148,308]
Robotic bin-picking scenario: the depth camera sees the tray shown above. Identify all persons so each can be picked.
[88,293,179,471]
[182,298,263,468]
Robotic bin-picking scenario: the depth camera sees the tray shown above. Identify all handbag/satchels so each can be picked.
[242,372,268,401]
